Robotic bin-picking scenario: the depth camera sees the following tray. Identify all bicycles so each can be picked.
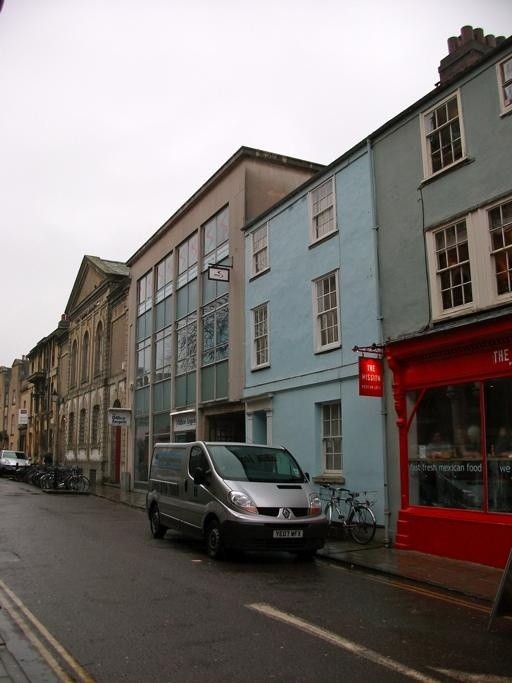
[14,461,92,494]
[318,476,376,547]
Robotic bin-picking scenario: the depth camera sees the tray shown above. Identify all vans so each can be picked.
[0,449,33,476]
[145,439,330,564]
[416,458,486,511]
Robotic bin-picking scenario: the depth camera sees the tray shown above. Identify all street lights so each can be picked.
[50,387,59,465]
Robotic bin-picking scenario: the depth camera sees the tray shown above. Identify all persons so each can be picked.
[462,425,484,459]
[426,425,453,461]
